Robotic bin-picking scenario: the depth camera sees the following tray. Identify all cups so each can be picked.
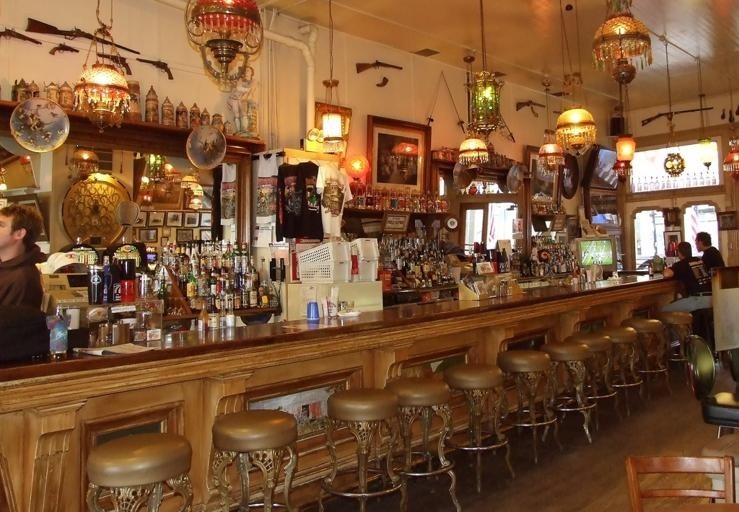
[326,296,339,317]
[341,300,354,313]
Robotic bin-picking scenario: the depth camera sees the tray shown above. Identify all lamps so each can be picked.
[590,0,654,85]
[183,0,264,93]
[555,1,597,150]
[457,49,487,166]
[662,42,685,176]
[721,89,739,174]
[72,0,136,131]
[466,0,501,134]
[320,109,346,145]
[613,3,636,177]
[538,82,562,170]
[698,62,714,168]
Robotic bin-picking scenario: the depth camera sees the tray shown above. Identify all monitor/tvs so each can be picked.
[581,145,619,190]
[574,237,618,278]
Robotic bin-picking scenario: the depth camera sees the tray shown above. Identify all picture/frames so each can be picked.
[314,1,354,163]
[365,114,432,197]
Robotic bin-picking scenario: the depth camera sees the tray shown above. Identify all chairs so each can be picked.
[686,335,739,440]
[625,456,736,510]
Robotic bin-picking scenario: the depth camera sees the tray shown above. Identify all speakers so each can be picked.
[610,116,624,137]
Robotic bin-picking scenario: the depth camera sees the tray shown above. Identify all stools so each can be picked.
[496,349,565,467]
[85,430,196,512]
[569,333,620,427]
[211,409,298,511]
[390,379,460,511]
[658,310,696,395]
[546,339,601,445]
[630,318,676,404]
[322,387,408,511]
[599,323,649,415]
[446,365,521,492]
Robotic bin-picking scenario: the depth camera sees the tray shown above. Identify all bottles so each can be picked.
[162,95,175,127]
[531,234,578,274]
[176,100,189,128]
[15,79,28,103]
[498,252,506,273]
[167,237,273,308]
[48,82,59,108]
[225,119,234,135]
[208,295,219,333]
[350,184,450,214]
[502,247,509,272]
[377,233,456,288]
[201,107,211,128]
[189,101,202,129]
[227,300,236,332]
[511,248,520,273]
[212,113,224,133]
[50,305,68,361]
[145,86,159,124]
[28,81,40,101]
[199,300,207,336]
[219,300,228,331]
[58,82,73,113]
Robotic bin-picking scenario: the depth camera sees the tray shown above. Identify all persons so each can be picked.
[0,203,43,365]
[695,232,724,277]
[663,242,719,362]
